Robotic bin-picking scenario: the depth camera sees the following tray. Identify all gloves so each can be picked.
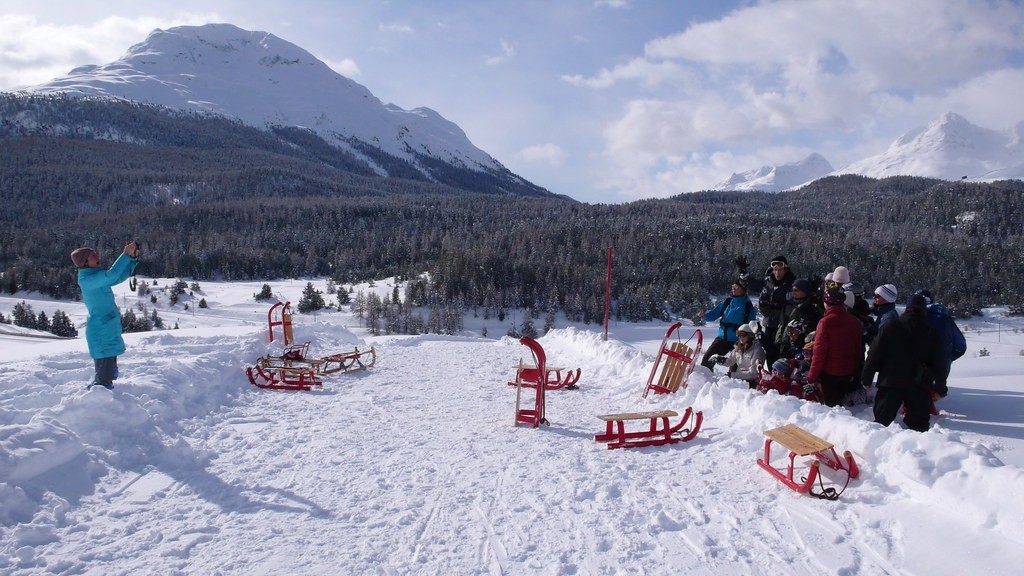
[717,357,726,365]
[735,255,751,276]
[803,384,812,392]
[724,372,731,378]
[805,331,816,343]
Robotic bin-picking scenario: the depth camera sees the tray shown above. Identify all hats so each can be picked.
[906,295,927,311]
[771,256,789,265]
[772,358,797,377]
[874,284,897,303]
[802,341,815,350]
[824,273,834,281]
[792,279,810,295]
[738,324,752,332]
[70,247,90,268]
[788,316,808,334]
[913,290,933,304]
[733,278,748,289]
[823,287,846,306]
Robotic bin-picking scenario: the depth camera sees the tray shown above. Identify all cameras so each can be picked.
[135,242,141,250]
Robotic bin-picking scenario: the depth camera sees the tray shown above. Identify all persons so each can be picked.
[698,255,967,432]
[71,242,139,389]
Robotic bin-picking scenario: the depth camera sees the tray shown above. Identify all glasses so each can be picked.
[738,331,750,338]
[873,295,881,299]
[770,261,784,269]
[802,352,812,355]
[788,331,797,335]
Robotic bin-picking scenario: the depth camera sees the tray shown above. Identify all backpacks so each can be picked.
[943,315,966,361]
